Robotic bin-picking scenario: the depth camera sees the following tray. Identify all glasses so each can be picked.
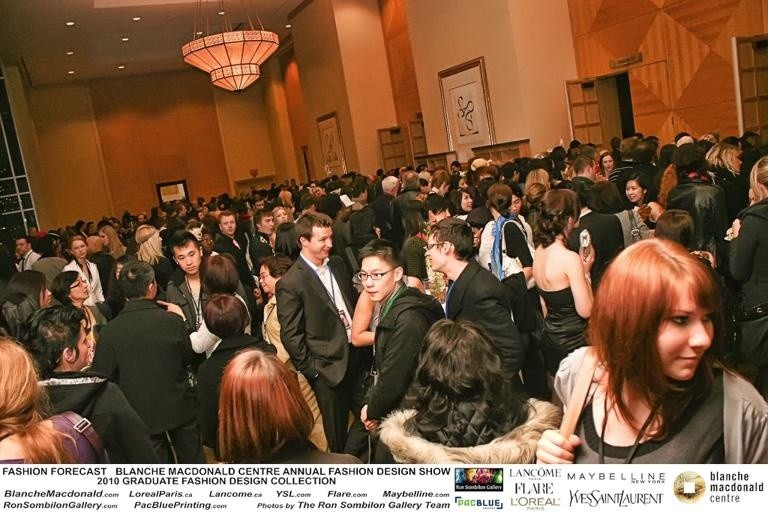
[426,242,445,250]
[357,265,400,281]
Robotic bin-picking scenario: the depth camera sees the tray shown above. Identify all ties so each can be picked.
[232,239,241,252]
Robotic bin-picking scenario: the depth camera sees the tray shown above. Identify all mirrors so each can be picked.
[155,179,189,205]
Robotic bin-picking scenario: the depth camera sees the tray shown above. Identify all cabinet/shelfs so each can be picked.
[470,137,532,164]
[411,151,460,175]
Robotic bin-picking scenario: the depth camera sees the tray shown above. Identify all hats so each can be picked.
[470,158,489,171]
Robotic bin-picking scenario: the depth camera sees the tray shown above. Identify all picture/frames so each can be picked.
[437,55,499,153]
[315,108,347,180]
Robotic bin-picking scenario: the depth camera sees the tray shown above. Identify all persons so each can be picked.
[1,132,768,463]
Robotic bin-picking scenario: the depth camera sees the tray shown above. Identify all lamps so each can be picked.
[179,0,281,97]
